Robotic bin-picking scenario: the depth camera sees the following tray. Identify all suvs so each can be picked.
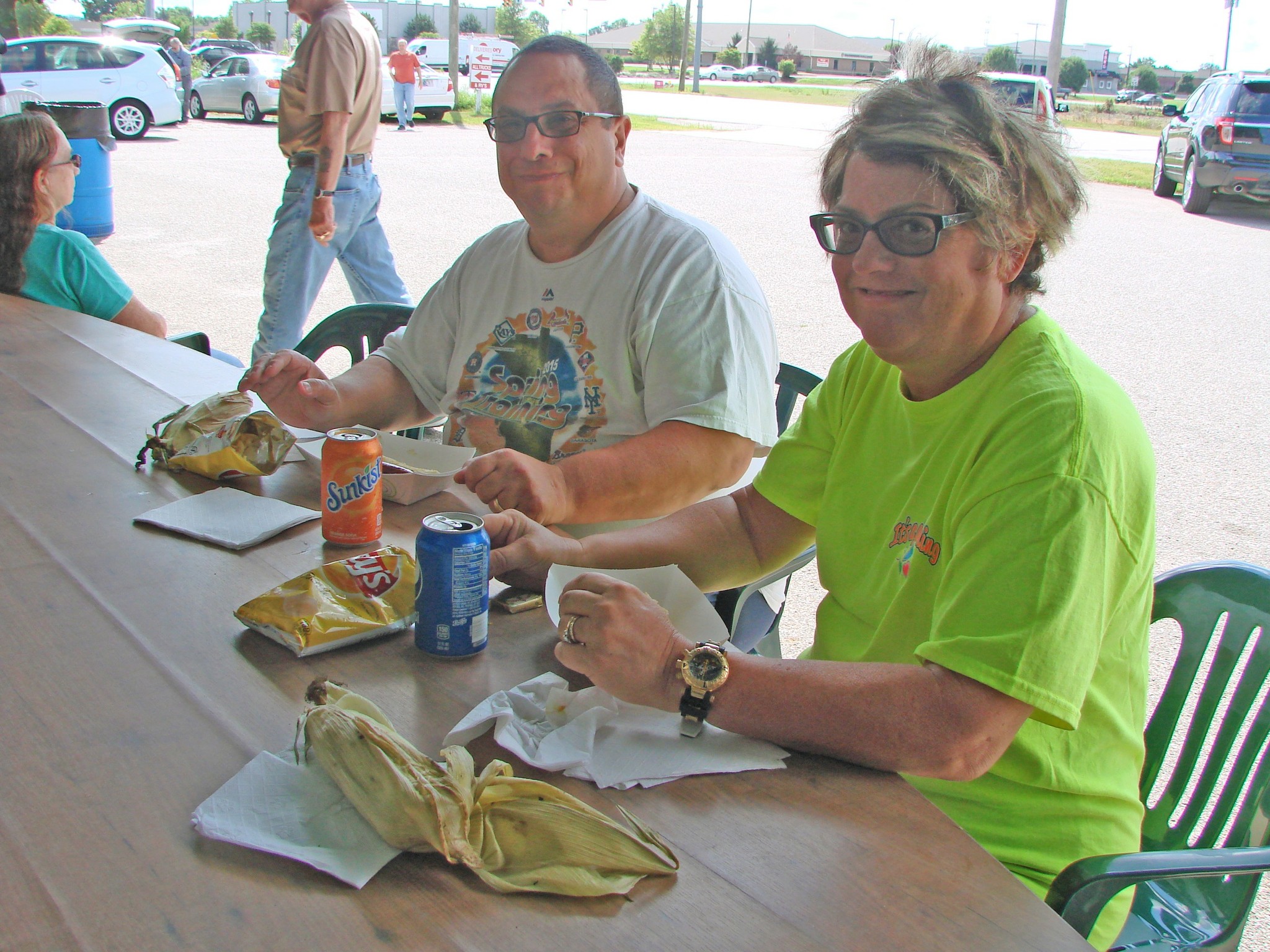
[1153,69,1270,214]
[189,38,277,60]
[972,72,1070,147]
[0,17,187,142]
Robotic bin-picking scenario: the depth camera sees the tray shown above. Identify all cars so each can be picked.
[1115,90,1146,103]
[731,65,780,82]
[699,65,739,81]
[190,54,294,124]
[1055,84,1073,100]
[375,56,455,124]
[189,46,243,73]
[1135,94,1163,107]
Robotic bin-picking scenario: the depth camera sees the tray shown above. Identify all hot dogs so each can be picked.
[381,454,440,474]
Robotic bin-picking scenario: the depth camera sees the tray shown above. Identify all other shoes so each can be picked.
[407,120,414,128]
[398,125,406,131]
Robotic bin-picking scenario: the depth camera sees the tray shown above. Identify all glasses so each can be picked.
[48,154,82,168]
[810,209,981,256]
[483,110,619,143]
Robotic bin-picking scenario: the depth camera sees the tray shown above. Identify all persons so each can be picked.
[237,35,789,658]
[1,1,424,365]
[483,37,1157,951]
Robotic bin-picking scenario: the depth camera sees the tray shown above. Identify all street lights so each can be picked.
[891,19,895,49]
[1223,0,1239,72]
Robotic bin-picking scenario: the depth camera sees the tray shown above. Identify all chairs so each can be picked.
[1047,561,1270,948]
[709,362,828,652]
[290,300,430,447]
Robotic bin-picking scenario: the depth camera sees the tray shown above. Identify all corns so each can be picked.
[293,677,476,869]
[152,388,253,461]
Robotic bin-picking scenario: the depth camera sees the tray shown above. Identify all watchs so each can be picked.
[674,638,730,738]
[314,187,334,199]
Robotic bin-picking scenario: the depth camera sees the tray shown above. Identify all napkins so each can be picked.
[441,671,794,791]
[131,485,323,551]
[190,748,409,891]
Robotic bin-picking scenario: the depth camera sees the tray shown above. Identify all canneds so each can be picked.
[322,428,383,548]
[414,512,488,659]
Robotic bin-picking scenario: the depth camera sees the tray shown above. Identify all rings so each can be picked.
[316,235,325,240]
[563,616,581,645]
[494,497,504,512]
[324,231,329,236]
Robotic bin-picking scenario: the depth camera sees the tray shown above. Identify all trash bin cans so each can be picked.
[21,101,116,237]
[654,80,663,89]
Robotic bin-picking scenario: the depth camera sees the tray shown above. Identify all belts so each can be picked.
[289,153,371,168]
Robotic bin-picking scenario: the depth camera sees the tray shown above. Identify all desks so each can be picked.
[0,291,1098,950]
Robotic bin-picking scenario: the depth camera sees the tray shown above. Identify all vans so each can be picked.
[406,38,450,73]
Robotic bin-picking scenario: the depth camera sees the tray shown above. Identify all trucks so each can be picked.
[459,35,522,75]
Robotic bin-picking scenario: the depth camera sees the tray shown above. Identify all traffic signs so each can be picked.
[469,45,492,89]
